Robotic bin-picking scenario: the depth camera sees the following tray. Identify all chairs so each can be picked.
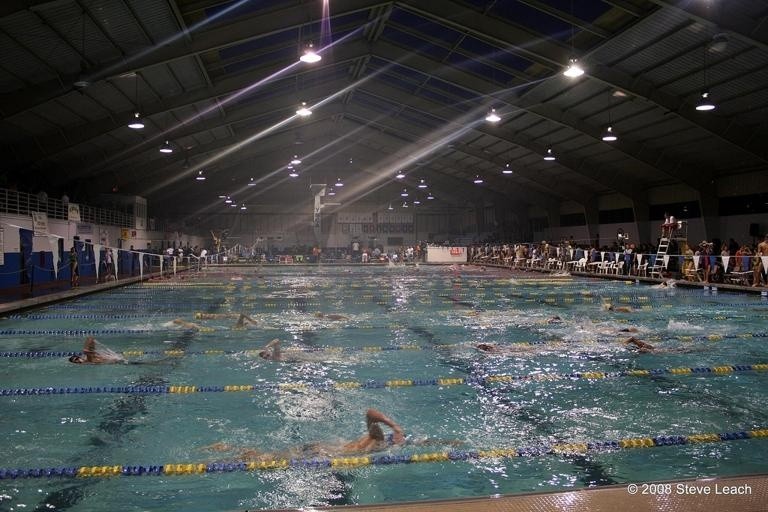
[471,243,650,278]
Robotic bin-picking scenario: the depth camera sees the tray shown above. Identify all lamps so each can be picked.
[543,119,556,161]
[158,111,173,153]
[501,142,514,173]
[561,54,585,77]
[73,6,92,87]
[193,152,485,210]
[695,24,716,112]
[126,70,145,130]
[485,105,501,122]
[601,85,617,143]
[299,42,323,63]
[296,101,312,118]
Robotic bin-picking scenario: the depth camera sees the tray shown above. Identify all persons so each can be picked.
[194,410,467,465]
[71,212,768,366]
